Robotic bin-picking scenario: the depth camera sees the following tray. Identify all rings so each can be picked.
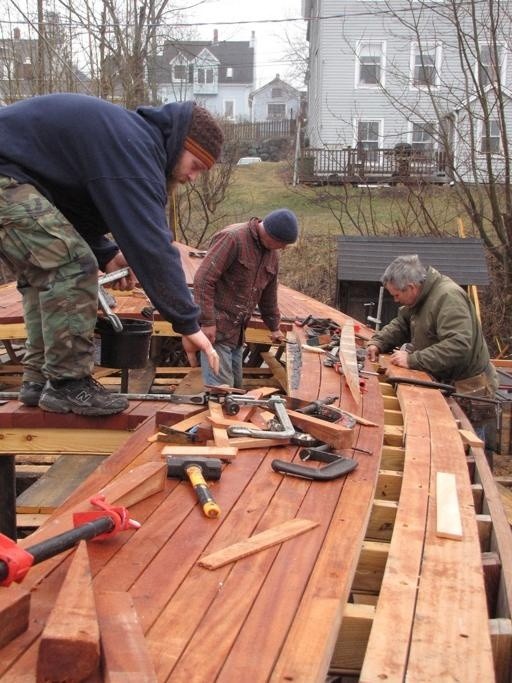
[210,348,217,355]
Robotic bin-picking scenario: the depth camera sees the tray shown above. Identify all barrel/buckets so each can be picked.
[95,317,154,367]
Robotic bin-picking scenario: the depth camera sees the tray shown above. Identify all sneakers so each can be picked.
[18,375,130,417]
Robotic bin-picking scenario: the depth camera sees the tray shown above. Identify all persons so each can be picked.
[1,90,221,417]
[363,253,500,450]
[193,206,300,395]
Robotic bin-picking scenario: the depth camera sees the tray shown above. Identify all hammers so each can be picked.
[167,455,222,519]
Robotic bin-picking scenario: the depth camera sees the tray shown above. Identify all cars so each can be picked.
[236,157,262,165]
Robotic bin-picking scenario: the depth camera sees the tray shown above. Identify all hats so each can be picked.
[263,211,297,244]
[184,105,222,170]
